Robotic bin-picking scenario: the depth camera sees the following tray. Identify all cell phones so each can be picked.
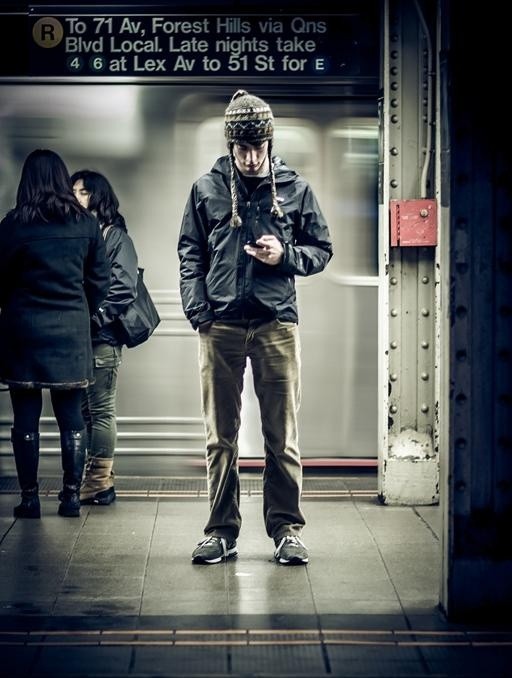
[247,241,264,248]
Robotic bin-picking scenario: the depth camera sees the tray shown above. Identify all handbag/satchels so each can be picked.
[108,266,161,347]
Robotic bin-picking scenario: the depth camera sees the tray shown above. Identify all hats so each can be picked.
[224,94,275,142]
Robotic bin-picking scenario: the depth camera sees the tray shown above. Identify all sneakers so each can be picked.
[191,530,238,564]
[274,535,310,566]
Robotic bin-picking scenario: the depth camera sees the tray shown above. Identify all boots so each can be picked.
[58,454,117,504]
[10,429,41,519]
[57,427,87,517]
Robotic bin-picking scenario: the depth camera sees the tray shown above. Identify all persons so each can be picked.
[2,148,114,519]
[66,167,161,509]
[174,90,336,568]
